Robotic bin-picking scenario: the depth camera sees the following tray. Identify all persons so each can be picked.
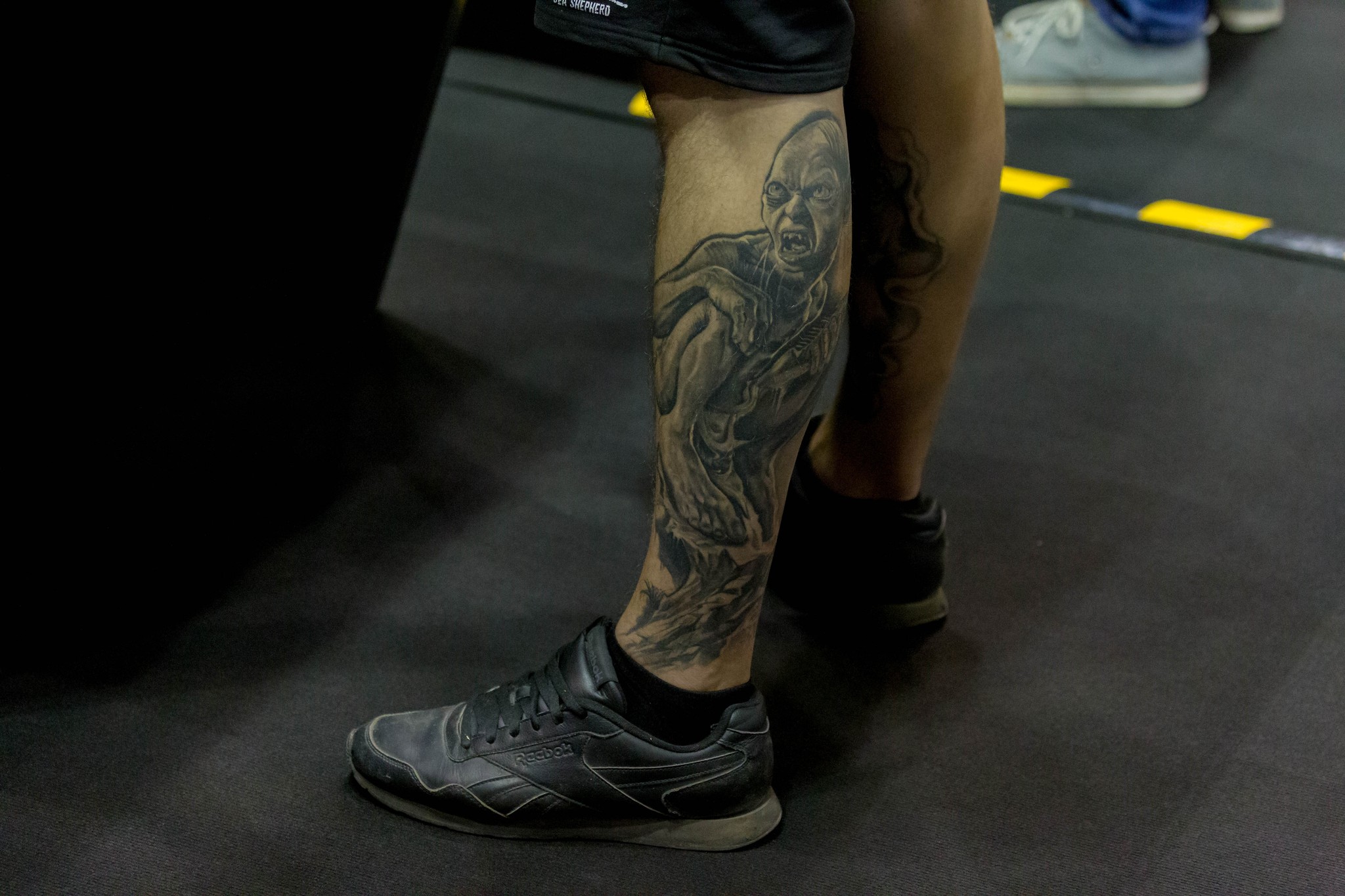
[344,0,1007,851]
[993,0,1287,109]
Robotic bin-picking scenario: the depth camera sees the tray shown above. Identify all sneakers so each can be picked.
[643,411,949,625]
[345,615,781,849]
[994,1,1282,105]
